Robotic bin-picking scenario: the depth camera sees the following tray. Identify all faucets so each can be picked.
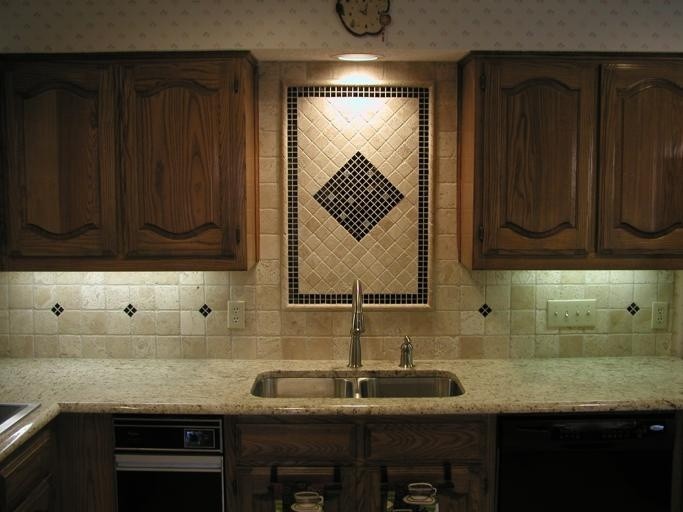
[346,279,366,367]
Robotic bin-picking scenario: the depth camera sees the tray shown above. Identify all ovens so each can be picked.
[499,415,676,512]
[109,413,230,512]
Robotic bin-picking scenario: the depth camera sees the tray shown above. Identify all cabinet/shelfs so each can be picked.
[223,416,497,512]
[458,50,683,271]
[0,51,257,273]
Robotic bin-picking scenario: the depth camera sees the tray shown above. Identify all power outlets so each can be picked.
[226,300,245,330]
[651,302,668,330]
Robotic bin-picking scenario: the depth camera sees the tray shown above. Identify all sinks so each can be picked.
[250,370,358,399]
[358,370,466,399]
